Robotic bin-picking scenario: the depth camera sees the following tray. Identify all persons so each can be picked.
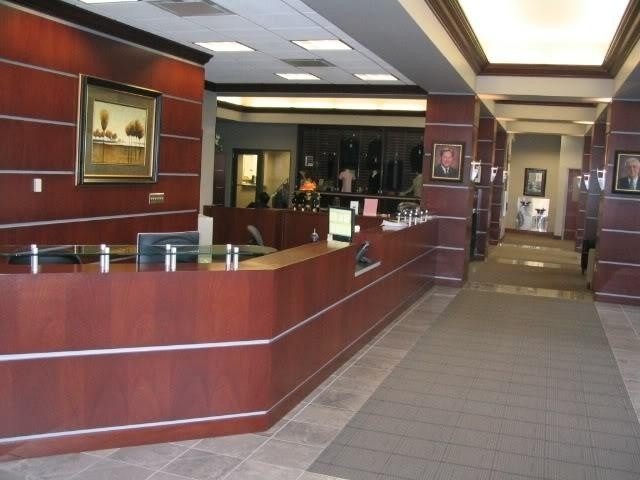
[398,167,422,198]
[337,168,358,193]
[245,191,271,210]
[434,148,459,178]
[366,169,380,194]
[617,157,640,191]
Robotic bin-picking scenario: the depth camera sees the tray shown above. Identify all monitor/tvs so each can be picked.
[136,230,199,264]
[328,206,355,242]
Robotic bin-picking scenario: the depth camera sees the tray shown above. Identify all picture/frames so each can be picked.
[611,149,640,195]
[75,73,163,188]
[523,168,547,197]
[430,141,466,183]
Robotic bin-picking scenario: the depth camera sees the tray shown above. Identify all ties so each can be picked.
[445,168,448,174]
[630,177,635,190]
